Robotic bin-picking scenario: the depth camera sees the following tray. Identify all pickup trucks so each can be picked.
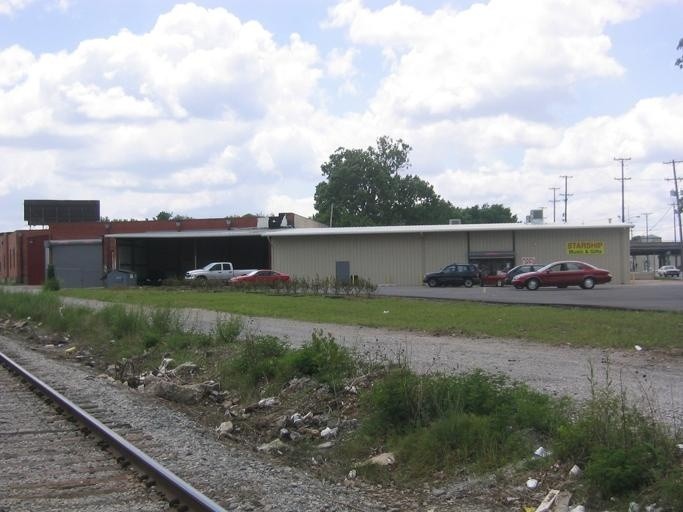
[183,262,257,288]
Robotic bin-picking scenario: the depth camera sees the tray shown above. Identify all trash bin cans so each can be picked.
[100,270,138,288]
[350,275,357,286]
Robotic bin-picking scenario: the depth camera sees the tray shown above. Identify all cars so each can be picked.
[657,266,679,277]
[510,260,611,291]
[422,264,479,288]
[479,269,508,286]
[504,264,553,286]
[229,270,289,286]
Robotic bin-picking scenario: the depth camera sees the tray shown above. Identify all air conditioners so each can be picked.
[449,219,461,224]
